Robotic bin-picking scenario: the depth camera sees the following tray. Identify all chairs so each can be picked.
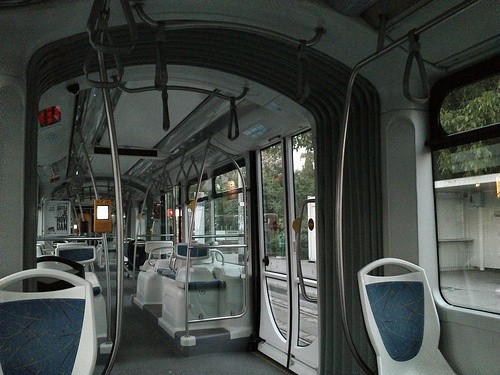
[0,268,99,375]
[29,229,226,295]
[357,256,459,375]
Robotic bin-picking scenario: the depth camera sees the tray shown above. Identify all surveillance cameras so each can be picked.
[67,83,80,95]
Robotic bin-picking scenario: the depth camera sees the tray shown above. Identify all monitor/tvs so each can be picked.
[96,205,109,220]
